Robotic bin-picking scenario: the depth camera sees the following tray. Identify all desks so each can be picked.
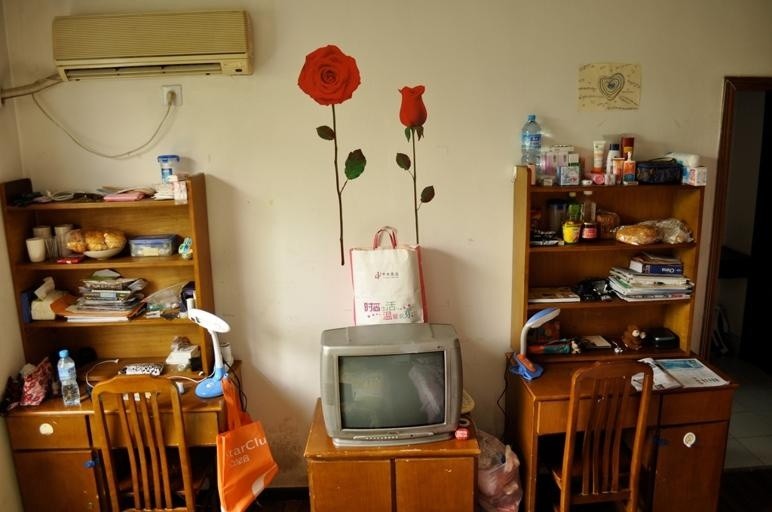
[503,349,741,512]
[3,359,246,512]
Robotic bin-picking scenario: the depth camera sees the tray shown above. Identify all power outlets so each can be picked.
[162,83,184,106]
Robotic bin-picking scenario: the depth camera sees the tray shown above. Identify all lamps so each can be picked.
[507,305,564,382]
[187,306,233,400]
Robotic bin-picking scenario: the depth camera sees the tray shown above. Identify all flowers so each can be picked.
[393,83,436,244]
[294,42,366,265]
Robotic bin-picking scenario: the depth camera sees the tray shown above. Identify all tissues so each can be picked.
[30,277,68,320]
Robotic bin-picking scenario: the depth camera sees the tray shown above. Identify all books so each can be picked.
[622,358,732,393]
[114,362,166,401]
[607,250,696,303]
[64,278,147,322]
[528,286,582,303]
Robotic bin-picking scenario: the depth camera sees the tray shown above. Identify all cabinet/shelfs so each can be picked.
[0,170,220,386]
[301,394,484,511]
[508,160,706,356]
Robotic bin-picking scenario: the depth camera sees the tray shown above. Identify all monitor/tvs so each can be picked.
[320,323,463,448]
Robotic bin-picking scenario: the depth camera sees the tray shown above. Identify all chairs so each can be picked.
[89,374,202,512]
[547,357,655,512]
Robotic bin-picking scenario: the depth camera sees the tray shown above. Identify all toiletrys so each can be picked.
[591,140,606,173]
[612,158,624,184]
[606,143,620,173]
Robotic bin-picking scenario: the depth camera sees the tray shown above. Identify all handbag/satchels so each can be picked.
[348,225,430,327]
[215,380,279,511]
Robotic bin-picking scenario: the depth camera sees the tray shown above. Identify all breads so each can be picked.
[65,227,126,253]
[615,224,657,245]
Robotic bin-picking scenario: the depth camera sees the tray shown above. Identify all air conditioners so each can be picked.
[51,6,258,85]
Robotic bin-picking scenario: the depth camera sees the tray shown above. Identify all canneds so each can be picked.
[581,222,598,241]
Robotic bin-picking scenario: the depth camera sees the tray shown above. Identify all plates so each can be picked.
[82,247,125,260]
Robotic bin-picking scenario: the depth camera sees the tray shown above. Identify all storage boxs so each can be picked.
[128,233,179,258]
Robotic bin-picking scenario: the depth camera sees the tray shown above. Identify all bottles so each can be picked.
[56,349,81,406]
[548,191,599,245]
[33,225,53,258]
[55,224,83,257]
[521,114,541,185]
[156,154,180,186]
[581,136,638,185]
[26,237,45,263]
